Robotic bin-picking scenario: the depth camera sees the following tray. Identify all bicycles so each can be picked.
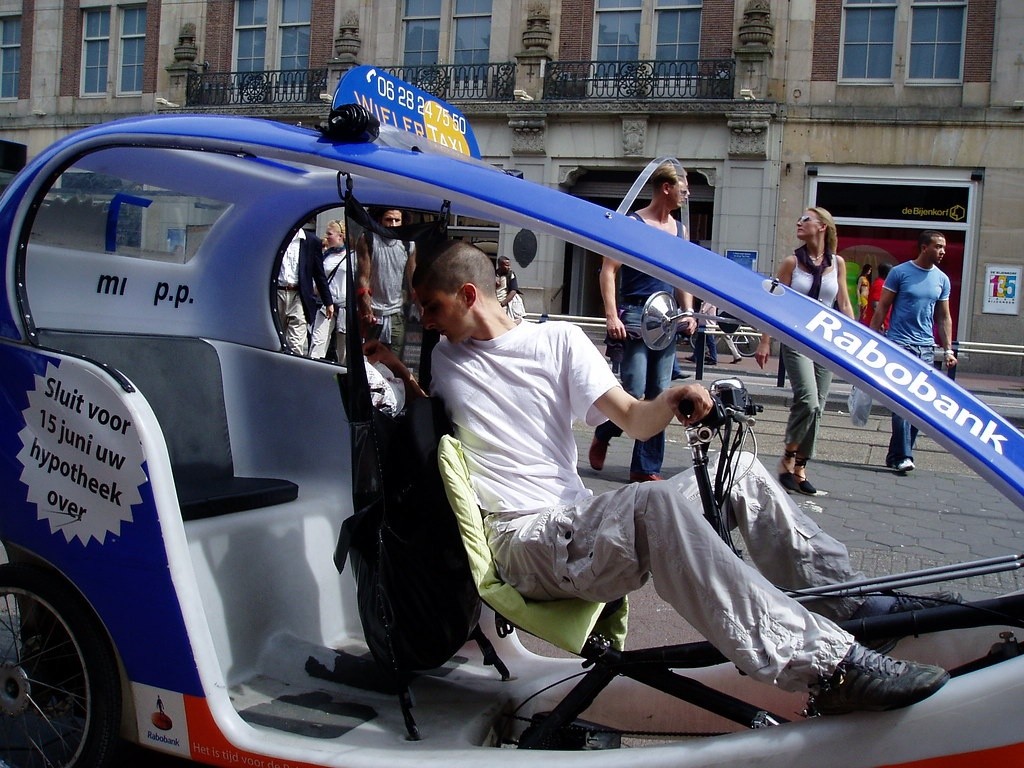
[688,323,763,358]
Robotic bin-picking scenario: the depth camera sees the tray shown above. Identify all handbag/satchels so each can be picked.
[332,496,482,679]
[846,379,872,426]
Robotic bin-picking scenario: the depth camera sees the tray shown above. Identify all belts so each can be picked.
[277,285,299,291]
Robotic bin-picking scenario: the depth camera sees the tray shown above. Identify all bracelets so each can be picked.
[944,350,954,356]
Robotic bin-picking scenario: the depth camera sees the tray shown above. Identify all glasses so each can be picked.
[797,216,819,223]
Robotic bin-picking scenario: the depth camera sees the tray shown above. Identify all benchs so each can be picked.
[24,334,335,680]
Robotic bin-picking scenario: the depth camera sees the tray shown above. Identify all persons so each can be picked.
[276,226,334,354]
[869,231,958,470]
[356,208,423,362]
[685,302,742,365]
[362,241,962,715]
[857,264,872,318]
[755,208,855,494]
[349,222,429,419]
[866,262,894,334]
[495,256,527,320]
[589,165,689,482]
[308,220,358,365]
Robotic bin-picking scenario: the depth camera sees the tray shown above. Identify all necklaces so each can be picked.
[810,252,825,260]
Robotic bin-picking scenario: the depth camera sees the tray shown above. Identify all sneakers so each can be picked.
[807,644,951,716]
[866,589,964,654]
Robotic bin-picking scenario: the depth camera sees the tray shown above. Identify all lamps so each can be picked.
[1014,100,1024,108]
[320,93,335,103]
[739,89,755,99]
[156,98,179,107]
[971,170,984,180]
[32,108,46,117]
[808,165,818,177]
[513,89,533,101]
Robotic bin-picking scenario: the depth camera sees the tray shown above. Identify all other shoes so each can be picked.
[631,468,663,484]
[684,355,697,362]
[672,372,692,381]
[730,355,743,363]
[794,472,818,498]
[885,456,917,471]
[777,461,802,494]
[707,358,717,367]
[589,431,610,470]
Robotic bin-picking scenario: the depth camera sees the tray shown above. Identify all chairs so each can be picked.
[437,433,664,659]
